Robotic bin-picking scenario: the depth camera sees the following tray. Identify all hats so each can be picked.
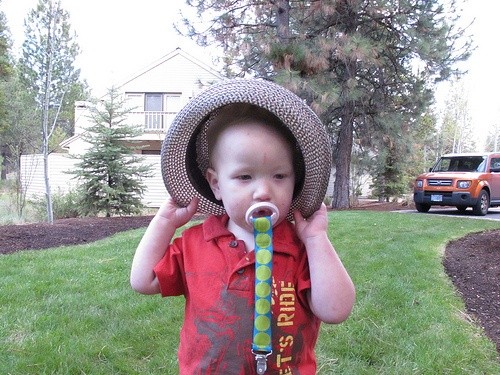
[161,77,332,222]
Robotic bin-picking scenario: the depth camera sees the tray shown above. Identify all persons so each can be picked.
[130,79,356,375]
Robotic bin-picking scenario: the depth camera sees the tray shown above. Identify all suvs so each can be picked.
[413,152,500,216]
